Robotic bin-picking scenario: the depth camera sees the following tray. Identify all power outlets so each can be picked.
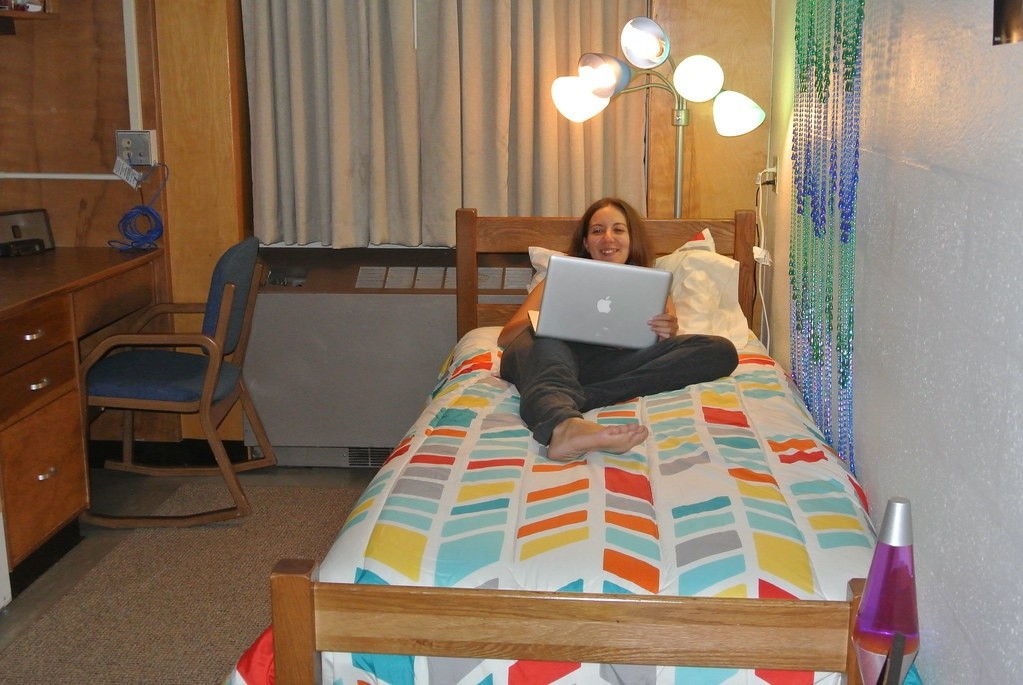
[770,156,780,193]
[116,129,157,165]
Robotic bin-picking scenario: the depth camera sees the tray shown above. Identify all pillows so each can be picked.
[527,241,751,352]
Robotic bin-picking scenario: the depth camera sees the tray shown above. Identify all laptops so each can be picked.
[527,254,673,349]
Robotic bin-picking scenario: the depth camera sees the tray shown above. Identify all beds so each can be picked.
[227,208,925,684]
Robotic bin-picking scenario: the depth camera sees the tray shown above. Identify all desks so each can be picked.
[0,239,167,577]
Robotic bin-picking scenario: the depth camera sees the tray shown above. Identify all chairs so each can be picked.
[81,234,270,526]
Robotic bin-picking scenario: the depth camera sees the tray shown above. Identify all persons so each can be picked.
[496,198,740,462]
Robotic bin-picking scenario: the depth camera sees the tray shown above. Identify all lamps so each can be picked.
[552,18,766,219]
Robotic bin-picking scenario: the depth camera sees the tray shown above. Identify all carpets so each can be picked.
[0,482,365,685]
